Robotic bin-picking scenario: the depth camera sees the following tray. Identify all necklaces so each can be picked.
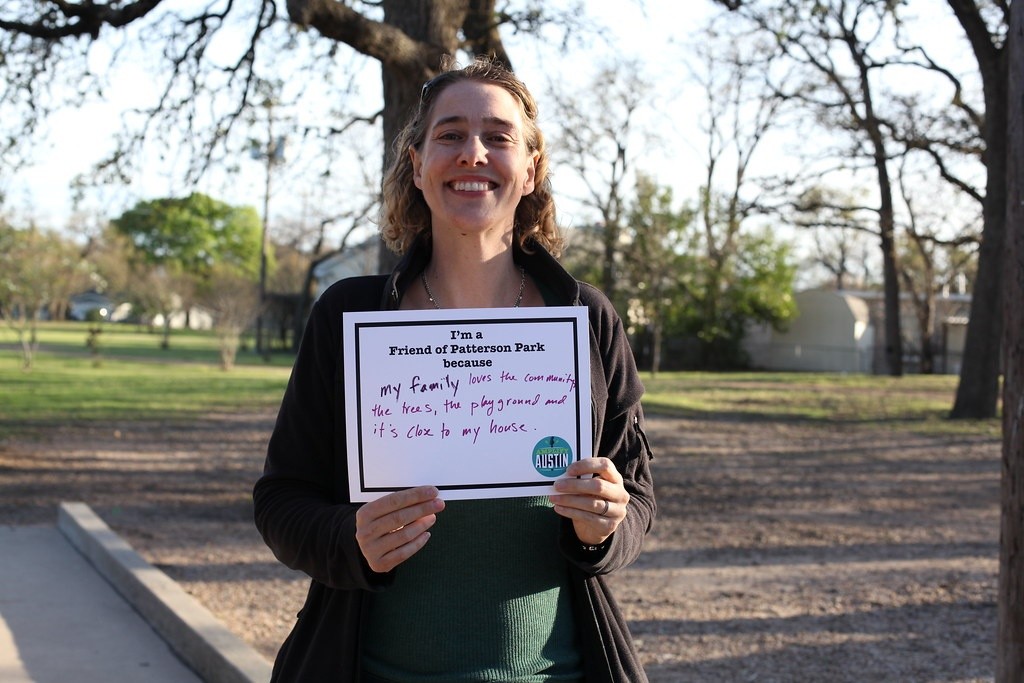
[421,266,527,309]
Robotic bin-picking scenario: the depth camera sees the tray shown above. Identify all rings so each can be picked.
[601,500,609,516]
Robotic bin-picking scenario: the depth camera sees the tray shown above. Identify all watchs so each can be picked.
[571,528,608,552]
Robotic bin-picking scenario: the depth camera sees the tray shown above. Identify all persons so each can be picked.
[253,61,657,683]
